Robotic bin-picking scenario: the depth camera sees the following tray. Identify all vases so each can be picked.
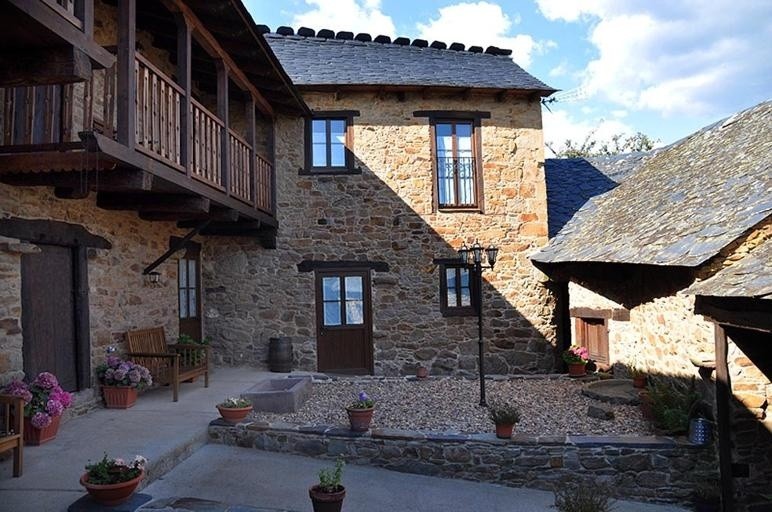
[10,414,61,445]
[99,385,138,409]
[632,374,661,418]
[217,402,253,422]
[80,466,144,505]
[346,406,375,432]
[568,362,586,374]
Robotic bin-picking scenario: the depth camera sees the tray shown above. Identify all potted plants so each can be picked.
[309,453,346,512]
[175,332,214,382]
[489,403,523,439]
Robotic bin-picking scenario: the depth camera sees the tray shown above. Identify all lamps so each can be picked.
[148,271,161,283]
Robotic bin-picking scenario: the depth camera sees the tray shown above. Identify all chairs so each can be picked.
[0,394,28,477]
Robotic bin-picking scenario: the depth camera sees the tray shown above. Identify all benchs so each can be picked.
[126,326,209,402]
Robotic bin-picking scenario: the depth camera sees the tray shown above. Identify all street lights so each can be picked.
[457,238,499,407]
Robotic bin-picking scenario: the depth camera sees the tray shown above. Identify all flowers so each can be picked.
[6,372,72,429]
[221,397,253,408]
[350,391,382,408]
[84,450,147,484]
[563,345,591,365]
[95,355,153,392]
